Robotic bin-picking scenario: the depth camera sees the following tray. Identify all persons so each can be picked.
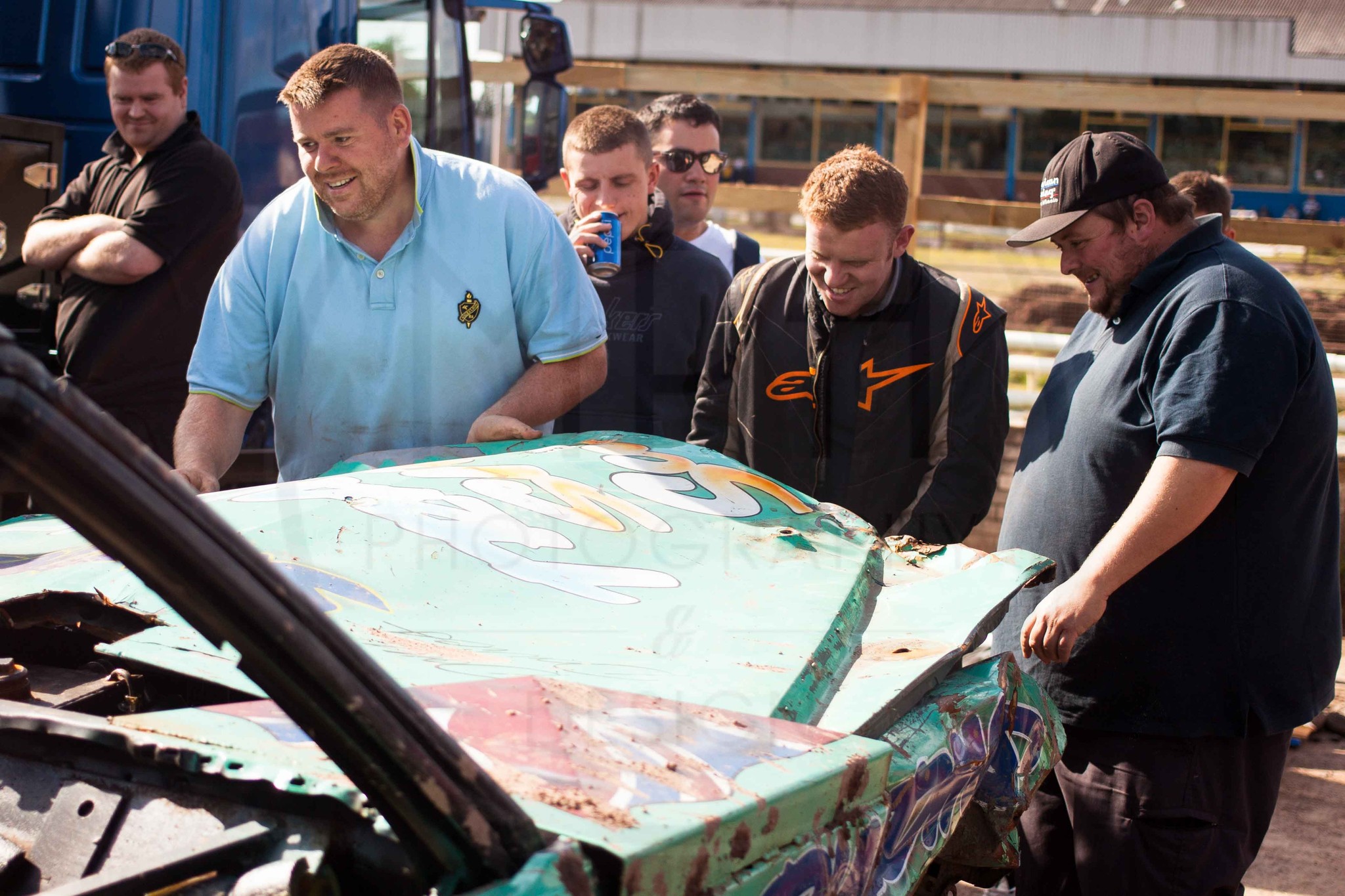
[687,143,1009,545]
[634,94,768,357]
[20,26,244,474]
[992,129,1298,896]
[536,102,735,450]
[1168,169,1242,248]
[168,41,608,498]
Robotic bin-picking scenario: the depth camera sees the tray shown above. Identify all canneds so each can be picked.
[586,211,621,277]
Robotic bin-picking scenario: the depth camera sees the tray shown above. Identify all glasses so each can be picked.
[654,148,729,175]
[104,41,184,67]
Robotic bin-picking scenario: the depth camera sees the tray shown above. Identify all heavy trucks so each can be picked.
[0,0,575,455]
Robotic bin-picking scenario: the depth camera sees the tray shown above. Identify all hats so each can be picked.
[1006,132,1169,248]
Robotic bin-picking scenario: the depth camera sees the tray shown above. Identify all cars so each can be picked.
[1,334,1090,896]
